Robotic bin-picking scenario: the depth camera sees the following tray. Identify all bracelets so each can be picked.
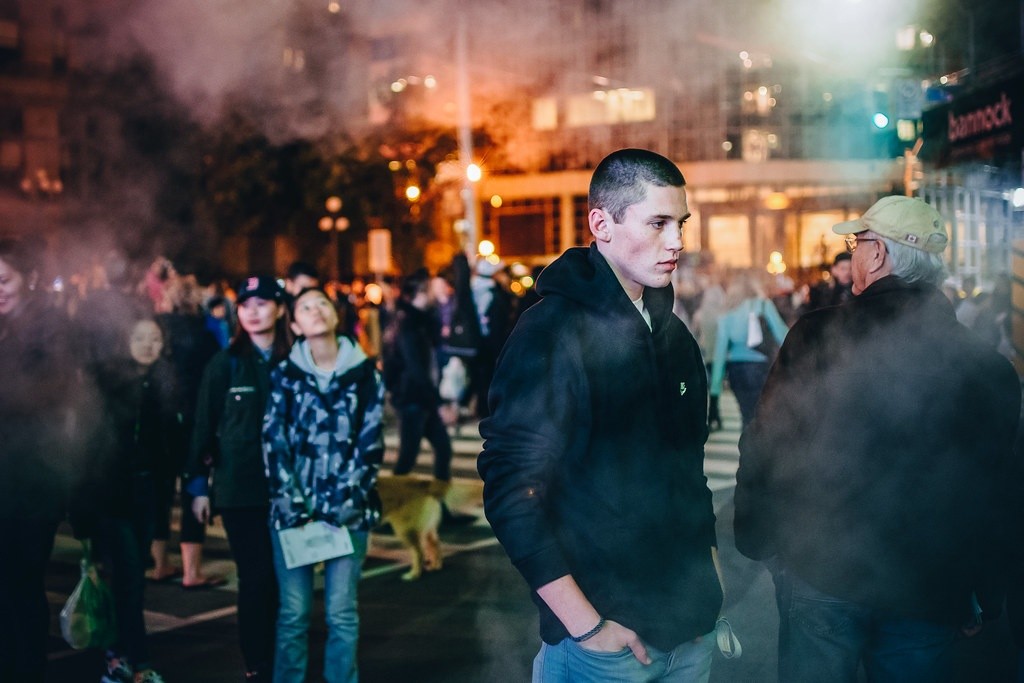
[570,616,605,643]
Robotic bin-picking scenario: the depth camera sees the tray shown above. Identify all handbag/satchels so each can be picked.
[748,297,776,358]
[59,559,117,652]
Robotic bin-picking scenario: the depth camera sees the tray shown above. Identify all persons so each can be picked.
[732,195,1024,683]
[477,148,734,683]
[0,251,1015,683]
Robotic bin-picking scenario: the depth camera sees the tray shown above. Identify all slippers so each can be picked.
[144,567,166,584]
[183,573,228,589]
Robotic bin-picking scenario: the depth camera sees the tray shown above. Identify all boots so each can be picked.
[430,477,452,502]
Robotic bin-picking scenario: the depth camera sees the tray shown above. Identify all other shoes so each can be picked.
[102,651,163,683]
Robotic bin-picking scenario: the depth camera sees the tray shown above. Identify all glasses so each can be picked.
[845,235,890,255]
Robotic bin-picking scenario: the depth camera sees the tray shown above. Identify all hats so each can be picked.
[236,274,281,303]
[831,196,948,253]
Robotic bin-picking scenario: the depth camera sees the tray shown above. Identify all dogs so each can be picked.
[313,475,484,581]
